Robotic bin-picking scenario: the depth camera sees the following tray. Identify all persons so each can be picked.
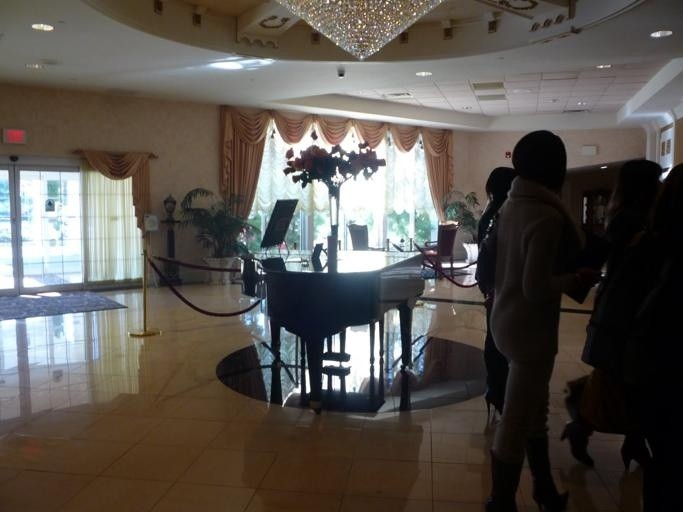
[475,131,683,512]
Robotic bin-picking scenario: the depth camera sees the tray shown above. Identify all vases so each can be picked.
[329,185,339,227]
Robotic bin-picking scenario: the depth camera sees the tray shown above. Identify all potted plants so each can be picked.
[181,189,261,285]
[440,190,481,264]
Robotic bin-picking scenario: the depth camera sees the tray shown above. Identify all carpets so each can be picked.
[0,290,128,321]
[423,267,470,278]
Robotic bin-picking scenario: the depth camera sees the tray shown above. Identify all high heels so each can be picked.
[621,435,651,471]
[560,419,594,466]
[485,391,504,415]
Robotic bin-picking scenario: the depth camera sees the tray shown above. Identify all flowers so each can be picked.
[282,142,386,201]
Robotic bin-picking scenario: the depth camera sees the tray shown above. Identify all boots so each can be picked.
[526,435,571,512]
[486,449,525,511]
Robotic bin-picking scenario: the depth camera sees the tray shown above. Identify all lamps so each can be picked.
[160,193,183,285]
[274,0,444,62]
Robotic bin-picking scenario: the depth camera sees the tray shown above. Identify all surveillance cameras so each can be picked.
[10,156,19,161]
[338,74,344,78]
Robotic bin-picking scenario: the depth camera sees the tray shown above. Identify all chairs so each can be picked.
[347,224,385,252]
[415,221,460,279]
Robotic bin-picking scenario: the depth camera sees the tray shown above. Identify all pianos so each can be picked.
[238,249,424,414]
